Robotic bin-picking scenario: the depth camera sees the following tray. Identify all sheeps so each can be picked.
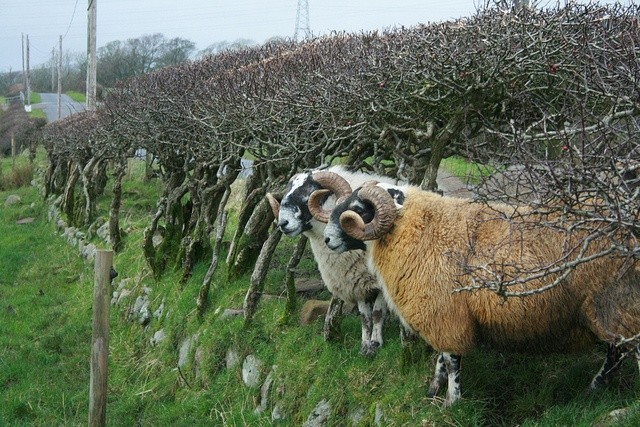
[265,157,639,411]
